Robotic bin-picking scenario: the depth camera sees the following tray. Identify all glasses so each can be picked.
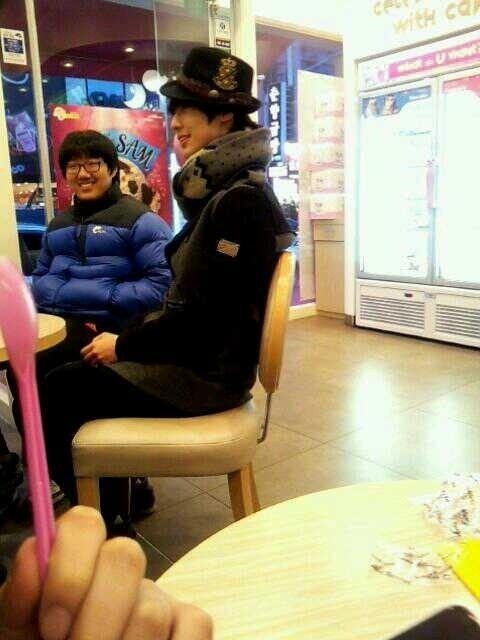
[65,161,101,174]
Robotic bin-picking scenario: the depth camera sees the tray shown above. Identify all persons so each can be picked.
[1,505,212,639]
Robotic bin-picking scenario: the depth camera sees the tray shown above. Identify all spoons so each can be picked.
[0,258,59,584]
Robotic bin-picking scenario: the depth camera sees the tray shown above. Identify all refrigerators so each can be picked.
[353,28,480,350]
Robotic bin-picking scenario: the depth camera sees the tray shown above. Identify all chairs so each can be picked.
[71,246,301,522]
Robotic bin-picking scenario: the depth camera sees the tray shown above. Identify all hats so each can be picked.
[159,46,261,113]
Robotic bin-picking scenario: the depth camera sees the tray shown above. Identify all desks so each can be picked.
[145,476,480,640]
[1,312,69,509]
